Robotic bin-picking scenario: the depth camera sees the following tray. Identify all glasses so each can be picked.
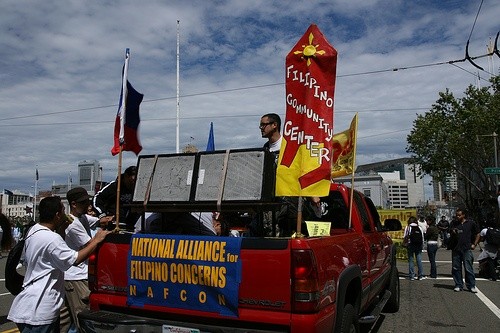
[259,122,274,129]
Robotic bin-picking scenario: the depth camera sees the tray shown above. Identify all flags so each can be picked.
[206,123,216,151]
[330,114,358,178]
[112,54,144,156]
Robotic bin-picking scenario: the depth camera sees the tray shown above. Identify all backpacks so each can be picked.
[5,224,56,296]
[484,227,500,249]
[410,225,423,250]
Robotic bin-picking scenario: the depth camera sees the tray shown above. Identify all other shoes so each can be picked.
[454,287,461,291]
[425,275,436,279]
[411,279,414,280]
[418,277,422,280]
[469,287,478,293]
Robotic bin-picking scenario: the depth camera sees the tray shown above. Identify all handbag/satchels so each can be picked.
[401,226,411,248]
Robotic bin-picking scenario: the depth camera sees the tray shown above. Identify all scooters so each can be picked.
[478,243,500,281]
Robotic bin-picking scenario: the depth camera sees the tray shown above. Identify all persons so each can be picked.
[0,165,500,333]
[258,113,321,237]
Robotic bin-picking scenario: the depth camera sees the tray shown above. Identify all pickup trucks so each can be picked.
[75,183,403,333]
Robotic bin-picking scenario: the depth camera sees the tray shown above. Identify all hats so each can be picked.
[66,187,93,203]
